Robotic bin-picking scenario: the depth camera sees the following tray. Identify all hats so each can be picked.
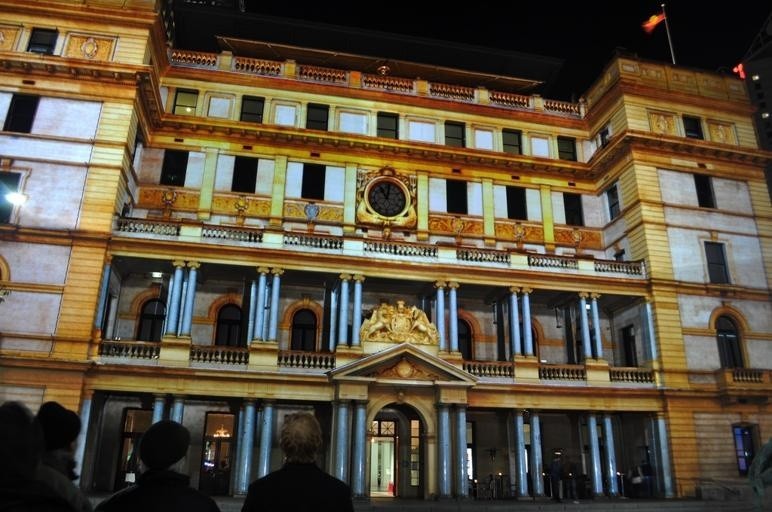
[140,419,190,469]
[35,401,81,451]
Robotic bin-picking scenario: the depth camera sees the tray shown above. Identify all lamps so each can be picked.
[149,270,165,278]
[3,185,29,208]
[550,444,567,458]
[212,423,231,440]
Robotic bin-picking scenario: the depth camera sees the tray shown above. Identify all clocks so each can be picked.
[356,163,418,230]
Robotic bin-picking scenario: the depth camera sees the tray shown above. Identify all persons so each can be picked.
[94,419,222,512]
[0,399,93,512]
[239,410,355,512]
[36,400,84,482]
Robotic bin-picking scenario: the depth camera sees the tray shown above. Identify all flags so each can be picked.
[642,12,666,32]
